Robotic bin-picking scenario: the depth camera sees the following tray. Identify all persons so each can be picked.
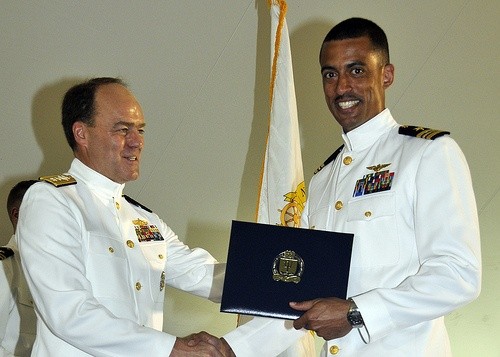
[0,181,37,357]
[187,16,483,357]
[16,78,226,357]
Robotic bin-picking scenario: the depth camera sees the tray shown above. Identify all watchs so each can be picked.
[346,299,364,328]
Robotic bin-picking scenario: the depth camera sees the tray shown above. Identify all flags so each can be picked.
[236,0,307,329]
